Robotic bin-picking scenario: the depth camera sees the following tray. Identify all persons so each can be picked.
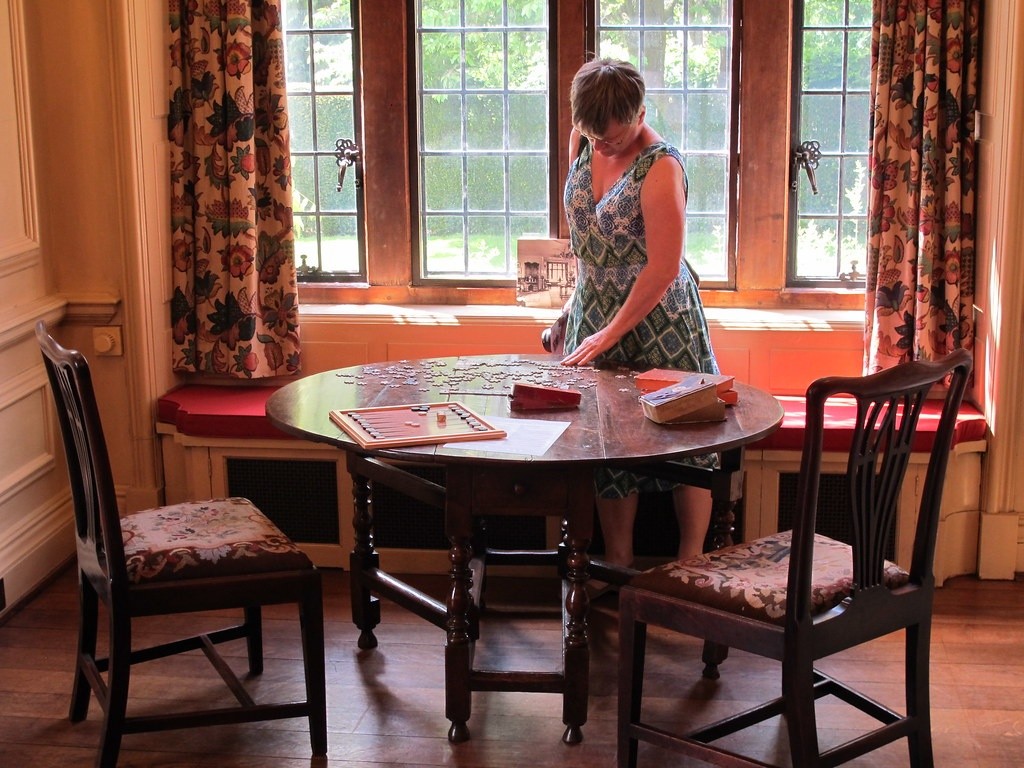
[541,55,724,602]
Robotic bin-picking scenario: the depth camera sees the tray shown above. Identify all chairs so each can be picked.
[34,319,327,768]
[617,348,973,768]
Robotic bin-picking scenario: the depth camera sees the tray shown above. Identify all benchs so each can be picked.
[152,382,987,589]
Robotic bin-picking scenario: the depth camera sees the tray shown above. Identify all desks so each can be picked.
[264,353,784,750]
